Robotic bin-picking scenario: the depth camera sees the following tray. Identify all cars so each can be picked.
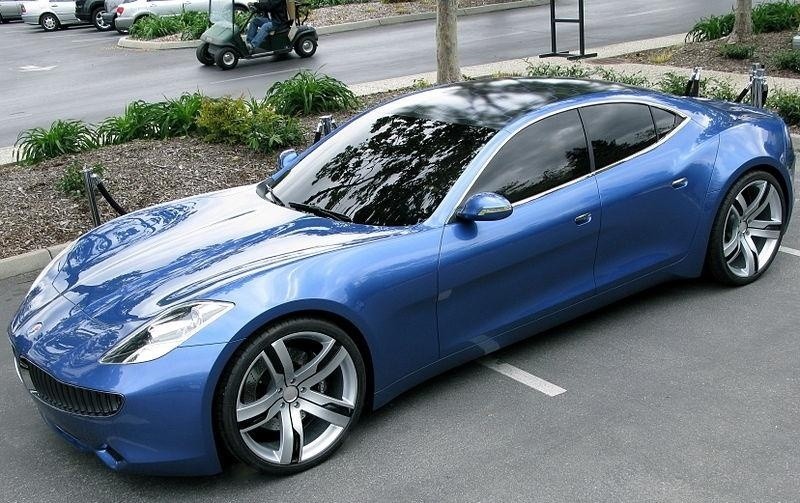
[6,67,797,482]
[0,0,260,32]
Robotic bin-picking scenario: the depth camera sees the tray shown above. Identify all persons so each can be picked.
[244,0,289,54]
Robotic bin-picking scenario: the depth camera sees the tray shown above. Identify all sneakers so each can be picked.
[246,43,255,55]
